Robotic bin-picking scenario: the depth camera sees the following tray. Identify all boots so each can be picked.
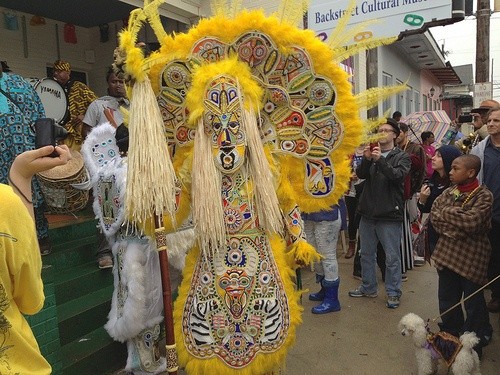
[345,239,357,258]
[309,274,325,301]
[311,277,341,314]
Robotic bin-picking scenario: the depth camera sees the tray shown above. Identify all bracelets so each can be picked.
[7,170,38,203]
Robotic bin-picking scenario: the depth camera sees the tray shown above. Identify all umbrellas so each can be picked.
[399,110,451,147]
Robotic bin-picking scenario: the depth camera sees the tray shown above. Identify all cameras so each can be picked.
[369,143,378,151]
[33,117,70,156]
[424,179,435,190]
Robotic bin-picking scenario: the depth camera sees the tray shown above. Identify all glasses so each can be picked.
[376,129,396,134]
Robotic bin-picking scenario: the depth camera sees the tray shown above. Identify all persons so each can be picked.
[114,0,412,375]
[0,58,168,375]
[284,98,500,358]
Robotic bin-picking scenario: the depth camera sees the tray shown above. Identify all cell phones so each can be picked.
[458,114,473,122]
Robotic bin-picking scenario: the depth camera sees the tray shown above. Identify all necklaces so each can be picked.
[472,133,479,149]
[450,184,482,210]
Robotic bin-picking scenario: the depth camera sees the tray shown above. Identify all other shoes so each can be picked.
[478,331,492,347]
[353,275,362,281]
[99,257,113,269]
[39,236,51,255]
[402,273,408,282]
[487,299,500,313]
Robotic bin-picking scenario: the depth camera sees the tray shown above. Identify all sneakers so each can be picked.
[385,296,400,309]
[348,286,377,298]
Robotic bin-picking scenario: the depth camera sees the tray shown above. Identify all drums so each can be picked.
[24,76,70,125]
[35,147,90,214]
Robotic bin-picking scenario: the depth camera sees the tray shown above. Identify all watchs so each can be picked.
[419,201,425,207]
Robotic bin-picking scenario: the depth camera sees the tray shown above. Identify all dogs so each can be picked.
[399,312,481,375]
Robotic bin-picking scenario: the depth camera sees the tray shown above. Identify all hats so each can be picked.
[471,99,500,113]
[384,118,400,138]
[53,60,71,71]
